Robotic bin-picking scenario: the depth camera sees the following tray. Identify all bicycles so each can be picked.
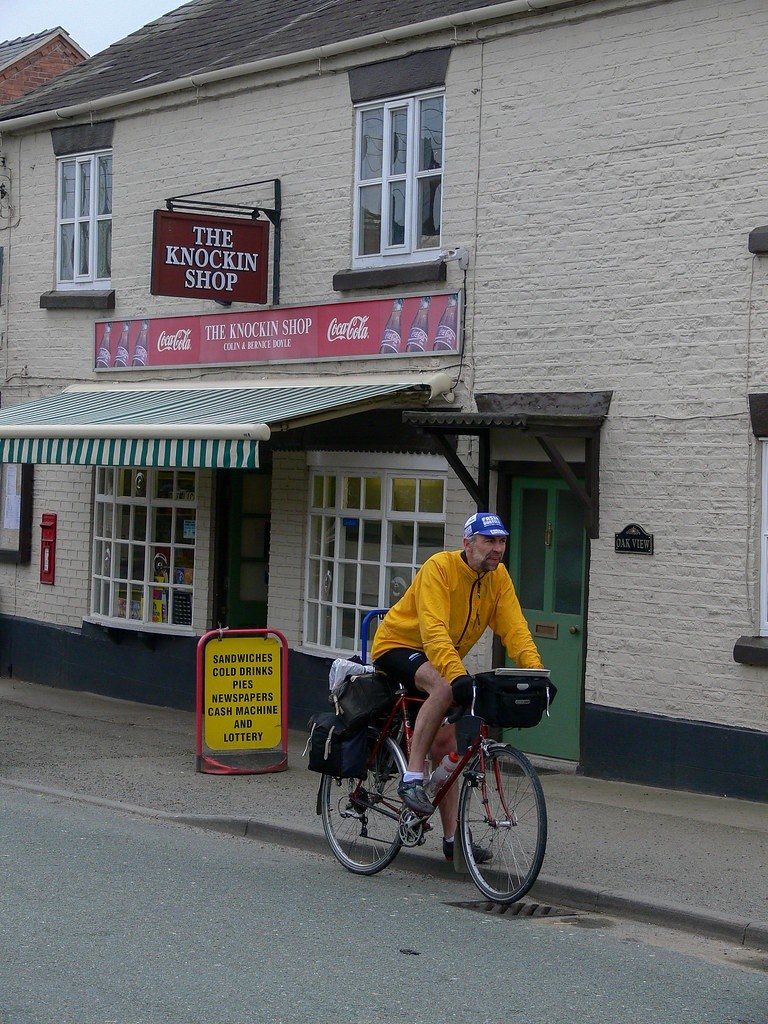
[317,661,548,905]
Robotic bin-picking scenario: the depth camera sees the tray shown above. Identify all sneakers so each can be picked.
[397,774,434,815]
[442,838,493,862]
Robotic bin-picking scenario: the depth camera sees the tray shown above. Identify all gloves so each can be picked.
[451,675,474,706]
[545,677,557,706]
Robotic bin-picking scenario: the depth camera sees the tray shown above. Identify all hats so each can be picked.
[464,513,509,538]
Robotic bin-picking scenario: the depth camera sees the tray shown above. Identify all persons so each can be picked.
[371,512,557,862]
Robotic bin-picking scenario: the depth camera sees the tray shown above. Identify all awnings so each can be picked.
[0,383,418,469]
